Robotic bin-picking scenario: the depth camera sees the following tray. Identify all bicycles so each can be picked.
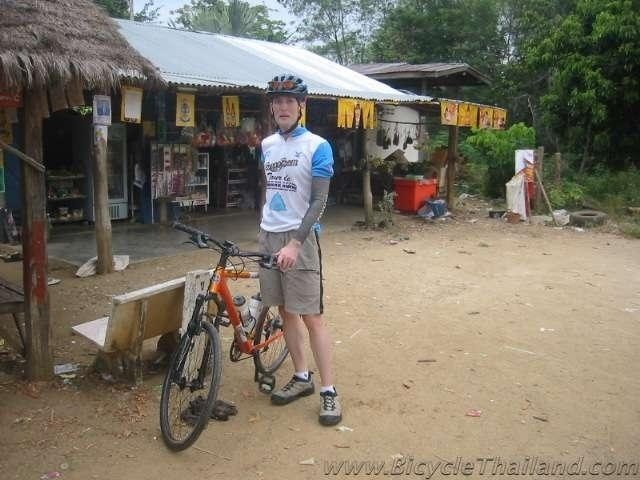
[157,219,295,453]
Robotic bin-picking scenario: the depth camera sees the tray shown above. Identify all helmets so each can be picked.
[263,74,307,101]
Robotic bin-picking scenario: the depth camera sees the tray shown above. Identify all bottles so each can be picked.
[233,295,251,328]
[249,292,263,321]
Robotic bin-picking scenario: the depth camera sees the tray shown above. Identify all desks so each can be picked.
[0,277,26,357]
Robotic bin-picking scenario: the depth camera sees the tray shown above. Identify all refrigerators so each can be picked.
[86,123,127,222]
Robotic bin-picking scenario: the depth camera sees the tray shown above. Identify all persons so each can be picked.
[253,73,342,427]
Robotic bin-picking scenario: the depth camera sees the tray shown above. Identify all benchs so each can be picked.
[70,267,233,380]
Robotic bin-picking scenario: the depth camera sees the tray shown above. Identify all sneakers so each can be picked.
[271,371,314,405]
[319,388,341,426]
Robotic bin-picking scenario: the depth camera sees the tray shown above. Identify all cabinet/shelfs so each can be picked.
[46,115,261,225]
